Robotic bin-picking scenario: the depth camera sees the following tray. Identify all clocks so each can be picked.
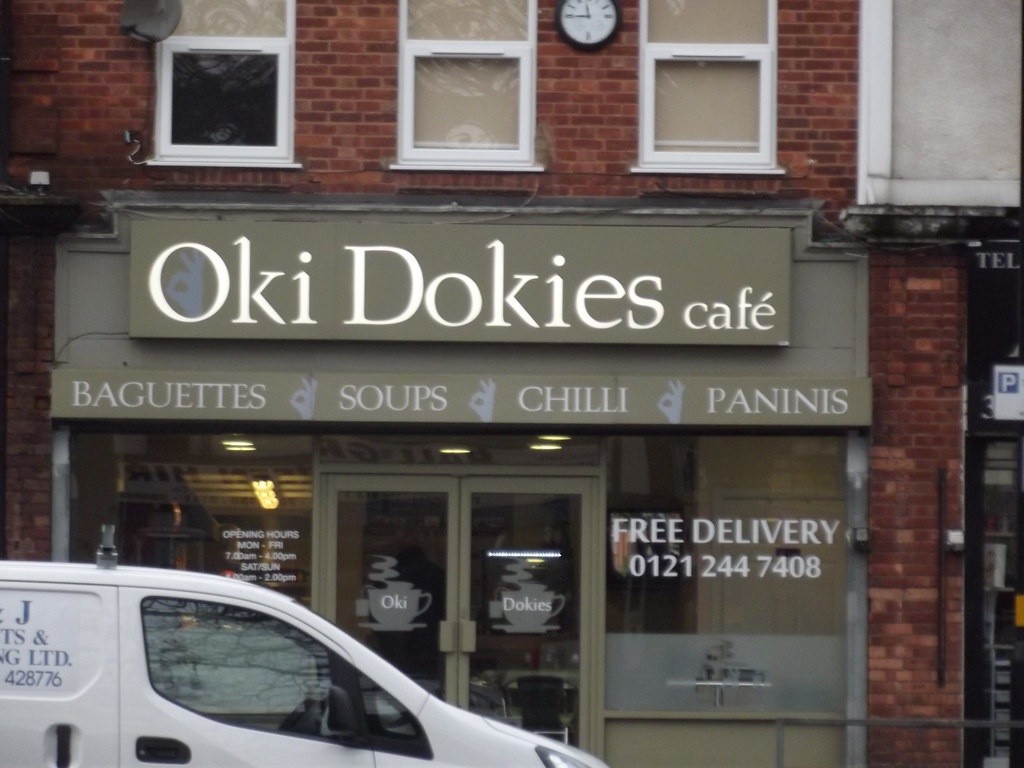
[555,0,622,49]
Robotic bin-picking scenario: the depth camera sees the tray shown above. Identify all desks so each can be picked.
[663,680,773,706]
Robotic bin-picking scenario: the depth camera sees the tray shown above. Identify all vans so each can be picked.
[0,557,611,768]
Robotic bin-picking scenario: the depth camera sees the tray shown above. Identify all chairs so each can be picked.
[517,674,577,750]
